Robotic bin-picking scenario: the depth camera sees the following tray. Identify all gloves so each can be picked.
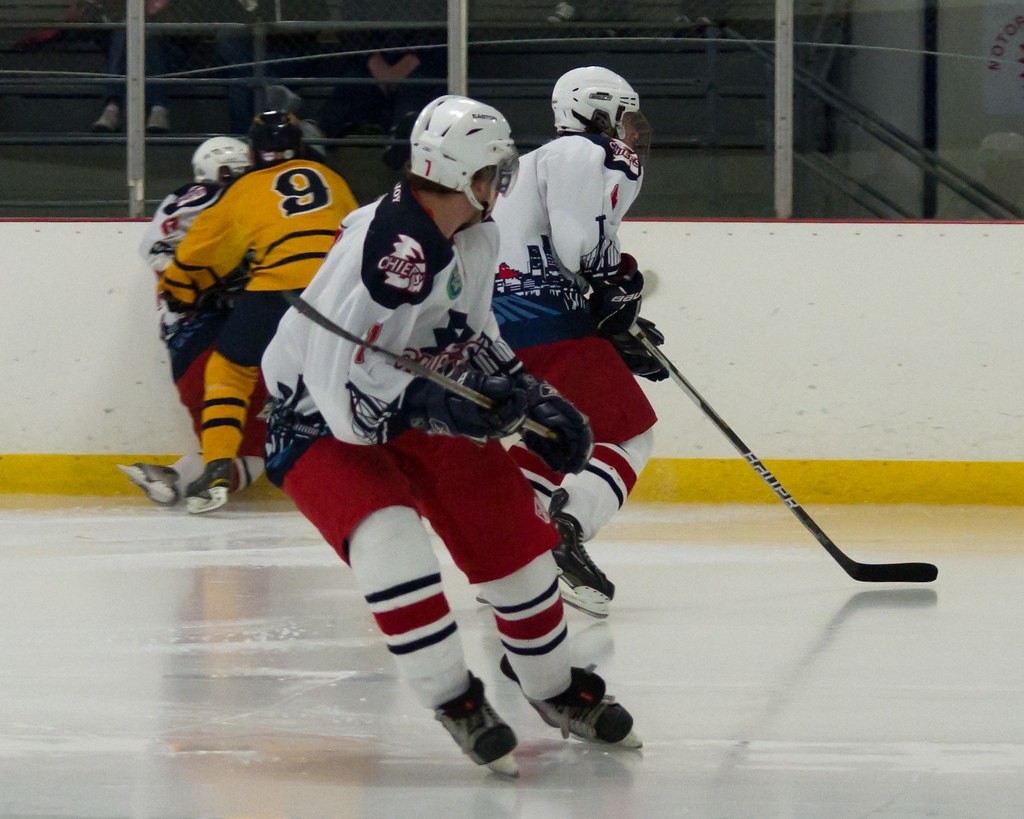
[610,316,667,383]
[390,377,528,445]
[470,340,591,473]
[589,254,642,334]
[156,298,188,342]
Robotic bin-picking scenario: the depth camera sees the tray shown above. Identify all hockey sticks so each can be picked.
[280,287,558,440]
[628,323,939,584]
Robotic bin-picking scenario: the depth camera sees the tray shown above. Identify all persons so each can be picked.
[128,137,266,502]
[157,112,359,514]
[63,0,328,133]
[263,94,642,776]
[293,0,448,161]
[490,65,670,620]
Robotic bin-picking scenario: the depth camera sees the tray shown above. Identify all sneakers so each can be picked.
[427,670,518,777]
[545,488,614,618]
[121,462,181,507]
[497,654,640,750]
[181,458,233,514]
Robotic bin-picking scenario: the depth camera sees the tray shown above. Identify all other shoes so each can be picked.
[146,109,169,131]
[92,110,120,132]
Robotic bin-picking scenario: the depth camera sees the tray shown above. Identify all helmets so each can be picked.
[191,135,254,185]
[408,95,517,194]
[550,68,639,135]
[247,110,306,152]
